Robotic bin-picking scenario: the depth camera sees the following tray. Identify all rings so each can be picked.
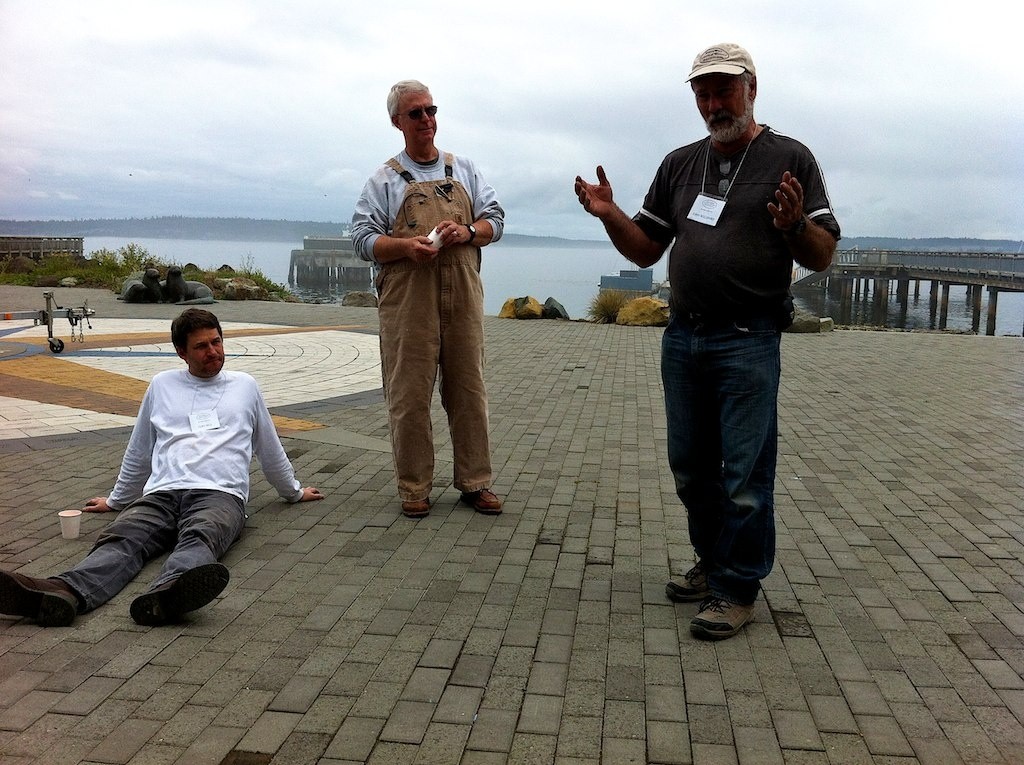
[454,231,457,235]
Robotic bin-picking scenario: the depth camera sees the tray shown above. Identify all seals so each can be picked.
[115,264,221,306]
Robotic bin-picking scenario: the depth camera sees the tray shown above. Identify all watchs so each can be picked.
[783,215,806,237]
[465,223,476,244]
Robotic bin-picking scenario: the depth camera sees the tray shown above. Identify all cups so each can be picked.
[58,510,82,539]
[425,226,447,250]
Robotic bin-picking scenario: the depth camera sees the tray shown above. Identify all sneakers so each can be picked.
[0,569,85,626]
[460,487,501,512]
[401,496,430,518]
[690,595,755,641]
[665,564,711,604]
[130,563,230,626]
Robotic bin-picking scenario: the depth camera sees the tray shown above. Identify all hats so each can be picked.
[685,43,756,83]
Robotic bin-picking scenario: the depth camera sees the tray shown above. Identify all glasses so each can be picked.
[395,105,437,121]
[719,159,732,195]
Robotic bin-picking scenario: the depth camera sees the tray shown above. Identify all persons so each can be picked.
[575,42,841,639]
[0,308,324,627]
[351,81,501,518]
